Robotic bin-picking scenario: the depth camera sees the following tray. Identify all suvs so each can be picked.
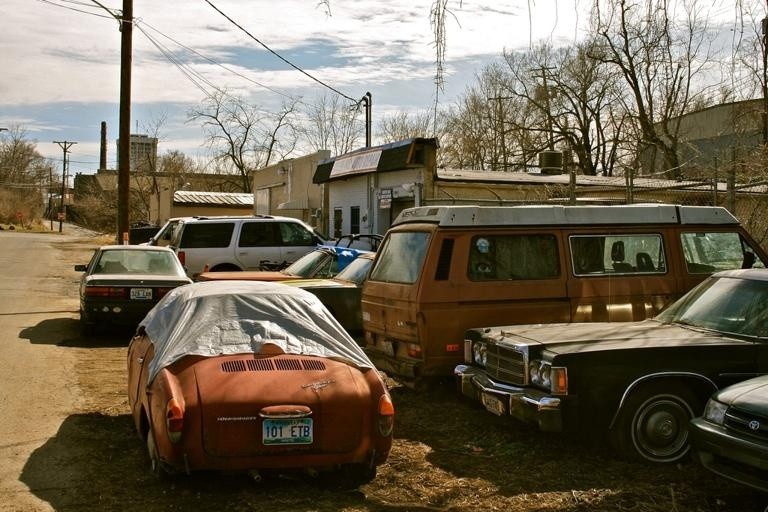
[167,213,337,281]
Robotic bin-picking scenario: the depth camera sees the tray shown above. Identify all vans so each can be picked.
[357,202,766,392]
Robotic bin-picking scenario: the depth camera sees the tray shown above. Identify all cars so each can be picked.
[126,280,395,484]
[686,373,768,498]
[455,268,768,467]
[196,246,374,282]
[73,245,192,328]
[137,218,180,250]
[281,253,378,345]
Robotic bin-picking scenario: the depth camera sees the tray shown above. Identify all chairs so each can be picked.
[612,240,654,272]
[102,257,166,273]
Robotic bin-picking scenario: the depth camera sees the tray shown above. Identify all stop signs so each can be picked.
[58,213,66,221]
